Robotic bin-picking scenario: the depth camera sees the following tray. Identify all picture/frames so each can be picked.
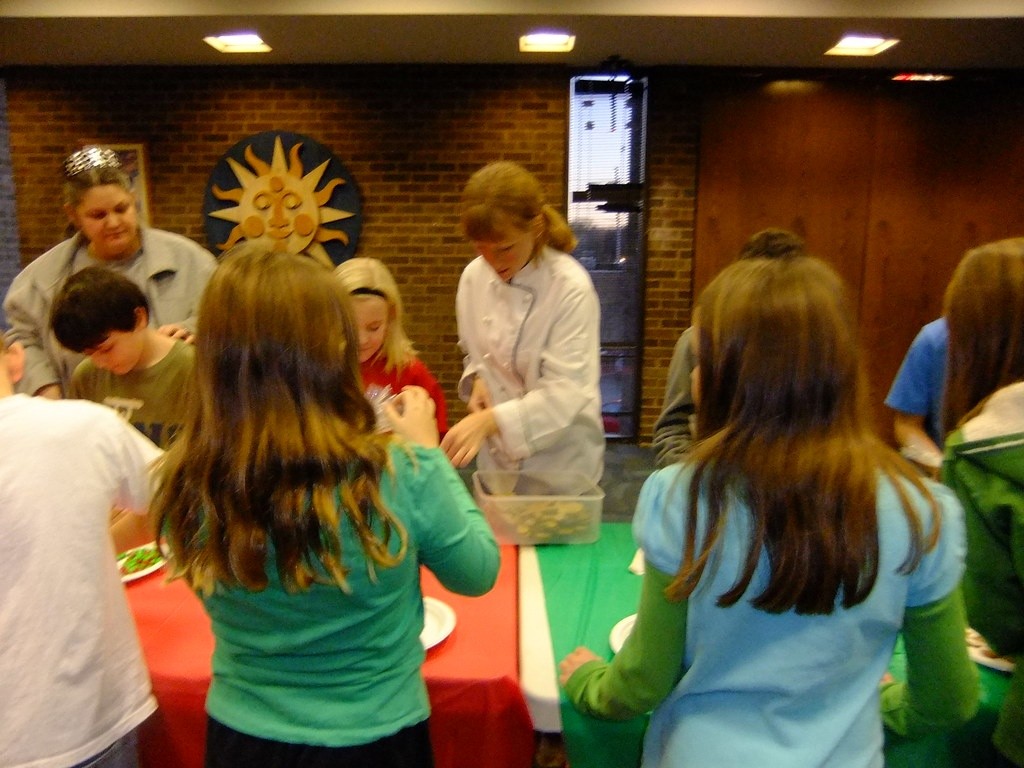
[80,143,149,229]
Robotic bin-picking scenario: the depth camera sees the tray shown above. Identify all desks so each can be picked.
[515,520,1009,768]
[115,507,533,768]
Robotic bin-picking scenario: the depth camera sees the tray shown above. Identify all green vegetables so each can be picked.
[116,548,161,573]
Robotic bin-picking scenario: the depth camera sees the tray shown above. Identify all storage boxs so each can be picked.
[474,468,605,543]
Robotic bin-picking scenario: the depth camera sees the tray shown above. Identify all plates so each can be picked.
[608,613,639,655]
[419,595,457,649]
[115,540,173,581]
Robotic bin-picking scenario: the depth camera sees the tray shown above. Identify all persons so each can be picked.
[561,256,981,768]
[437,161,605,496]
[49,267,197,447]
[147,243,501,768]
[652,229,809,464]
[0,147,217,399]
[937,236,1024,768]
[333,256,448,437]
[0,322,168,768]
[884,317,951,463]
